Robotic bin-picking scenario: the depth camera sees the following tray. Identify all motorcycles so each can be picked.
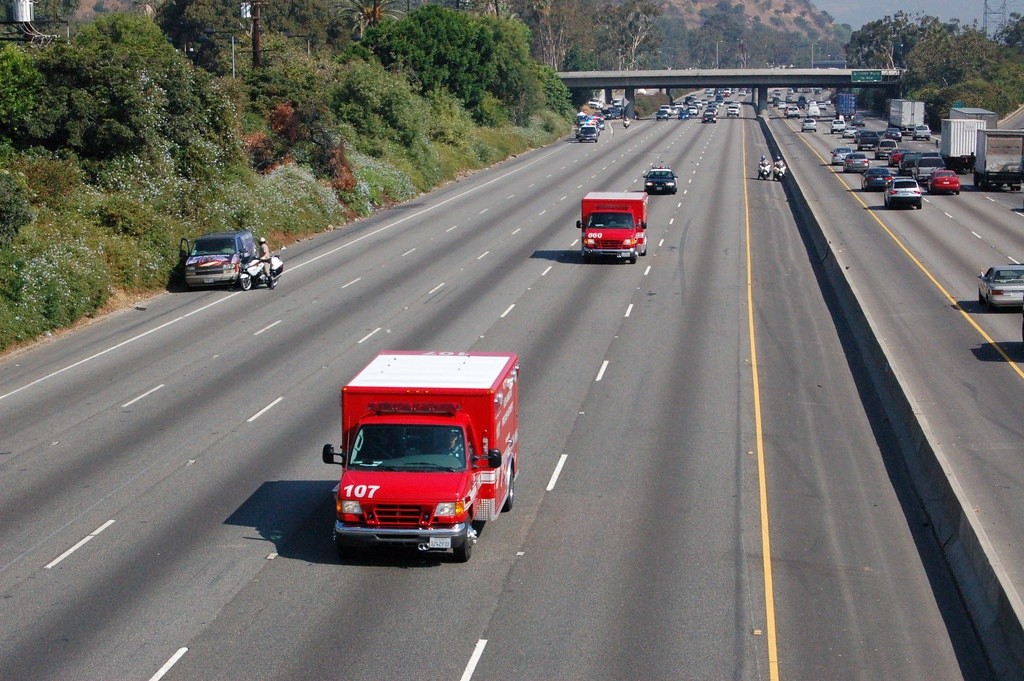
[237,252,284,292]
[757,162,772,180]
[773,163,787,182]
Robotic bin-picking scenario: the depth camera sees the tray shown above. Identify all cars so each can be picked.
[925,170,960,196]
[883,128,903,142]
[655,86,856,124]
[643,165,678,194]
[830,146,855,166]
[573,90,629,143]
[841,153,870,174]
[841,126,858,138]
[851,116,866,127]
[860,167,892,192]
[854,129,870,144]
[977,264,1024,312]
[921,152,940,157]
[887,148,915,167]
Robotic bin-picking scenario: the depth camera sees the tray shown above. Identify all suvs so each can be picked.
[800,118,817,132]
[829,120,846,134]
[872,139,898,160]
[884,177,923,210]
[898,152,925,177]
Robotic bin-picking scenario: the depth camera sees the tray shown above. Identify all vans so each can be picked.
[179,231,256,290]
[912,156,947,186]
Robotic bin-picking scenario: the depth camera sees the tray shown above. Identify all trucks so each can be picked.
[935,119,987,173]
[835,93,856,120]
[969,128,1024,192]
[886,99,925,136]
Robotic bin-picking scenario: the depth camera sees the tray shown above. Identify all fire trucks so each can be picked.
[575,191,650,264]
[323,349,519,563]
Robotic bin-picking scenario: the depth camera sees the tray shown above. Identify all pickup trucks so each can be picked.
[856,131,881,151]
[912,125,932,141]
